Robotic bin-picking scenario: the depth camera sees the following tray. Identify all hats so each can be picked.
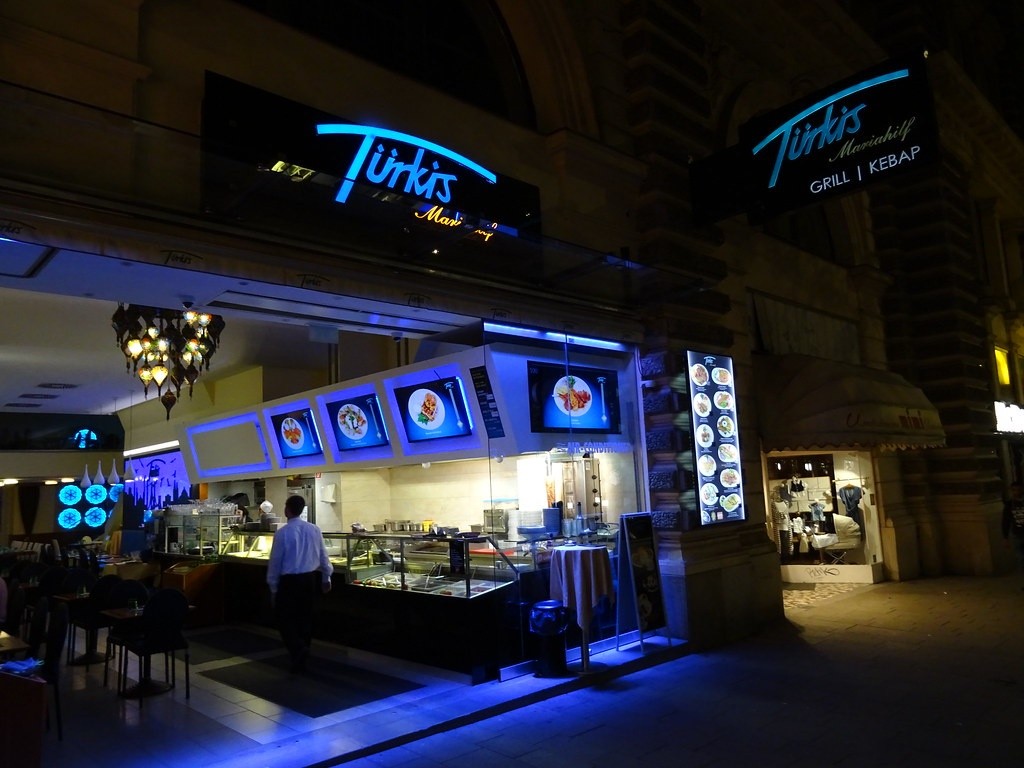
[80,536,94,545]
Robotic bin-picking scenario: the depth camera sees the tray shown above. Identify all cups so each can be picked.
[128,601,138,613]
[78,585,86,597]
[170,542,176,551]
[562,519,573,537]
[30,576,37,585]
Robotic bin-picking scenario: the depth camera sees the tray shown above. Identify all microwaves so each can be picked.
[484,509,512,532]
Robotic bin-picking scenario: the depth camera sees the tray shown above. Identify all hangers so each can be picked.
[771,495,788,504]
[844,479,854,489]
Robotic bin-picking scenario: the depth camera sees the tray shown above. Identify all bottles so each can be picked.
[576,502,584,536]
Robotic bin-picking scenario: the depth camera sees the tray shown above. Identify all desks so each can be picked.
[0,630,30,659]
[814,543,856,565]
[0,550,37,560]
[67,556,162,589]
[100,604,196,700]
[51,592,114,666]
[549,545,615,675]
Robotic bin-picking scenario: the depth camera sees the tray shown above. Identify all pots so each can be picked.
[373,520,423,531]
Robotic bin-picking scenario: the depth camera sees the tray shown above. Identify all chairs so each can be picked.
[0,674,48,768]
[7,559,38,640]
[65,575,124,669]
[28,543,44,584]
[123,588,190,709]
[38,562,78,658]
[36,604,69,741]
[10,540,23,560]
[102,579,169,697]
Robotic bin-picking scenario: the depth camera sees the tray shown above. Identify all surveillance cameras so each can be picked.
[181,298,194,309]
[392,332,403,342]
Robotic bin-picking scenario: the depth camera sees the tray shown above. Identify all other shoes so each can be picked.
[289,646,311,674]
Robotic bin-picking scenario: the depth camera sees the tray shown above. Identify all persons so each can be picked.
[264,495,335,675]
[1000,481,1024,550]
[789,474,806,497]
[772,497,794,565]
[79,535,100,573]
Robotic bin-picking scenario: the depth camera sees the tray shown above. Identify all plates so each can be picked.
[282,418,304,449]
[717,416,734,437]
[697,425,714,447]
[698,454,716,476]
[720,469,740,487]
[692,364,710,386]
[719,494,741,512]
[554,376,592,417]
[711,368,731,385]
[694,393,711,417]
[701,483,719,522]
[718,445,738,462]
[408,389,445,430]
[462,532,480,538]
[713,391,733,409]
[337,404,368,440]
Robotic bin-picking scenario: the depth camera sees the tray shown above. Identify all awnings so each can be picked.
[757,351,949,454]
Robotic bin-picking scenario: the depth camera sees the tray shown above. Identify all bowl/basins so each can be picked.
[543,508,559,536]
[517,526,546,538]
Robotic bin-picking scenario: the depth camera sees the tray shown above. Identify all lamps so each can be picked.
[80,408,92,488]
[123,390,136,483]
[93,401,105,485]
[110,302,226,421]
[108,395,120,486]
